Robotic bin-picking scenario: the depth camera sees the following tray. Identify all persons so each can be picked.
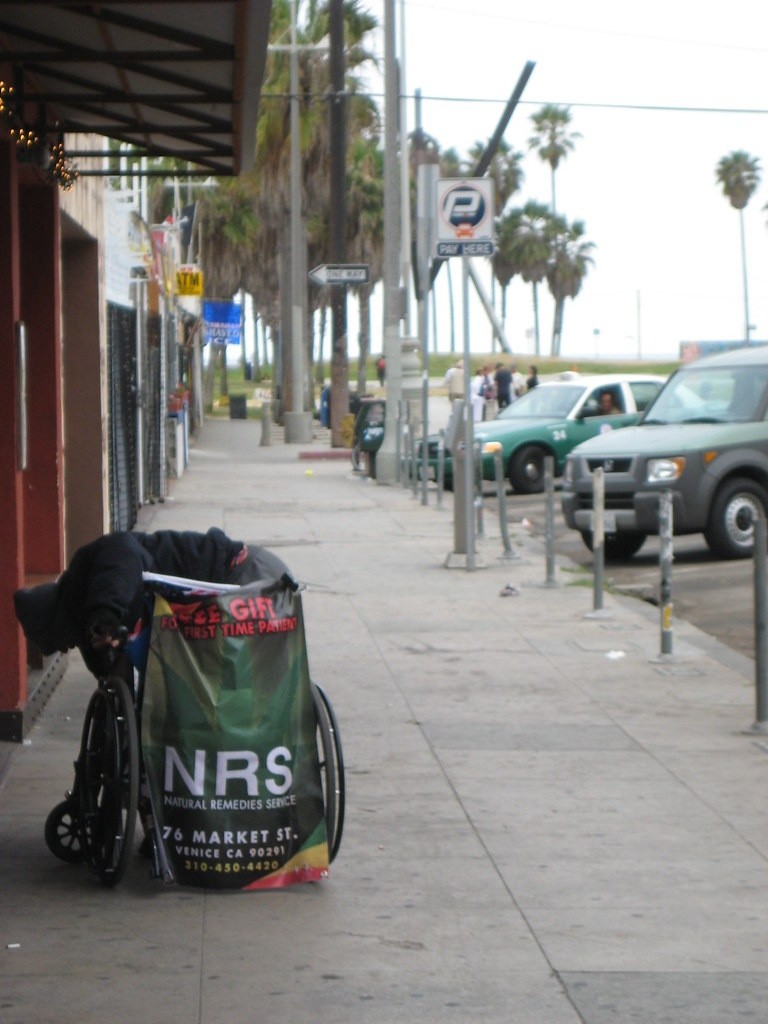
[442,358,539,423]
[579,392,620,419]
[375,354,385,386]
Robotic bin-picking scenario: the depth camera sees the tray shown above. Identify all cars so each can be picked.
[401,369,732,494]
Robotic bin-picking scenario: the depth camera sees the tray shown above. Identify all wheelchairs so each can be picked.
[42,529,348,889]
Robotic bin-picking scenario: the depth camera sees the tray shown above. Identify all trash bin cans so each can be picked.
[228,395,247,419]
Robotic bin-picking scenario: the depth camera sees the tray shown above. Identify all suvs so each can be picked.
[561,344,768,562]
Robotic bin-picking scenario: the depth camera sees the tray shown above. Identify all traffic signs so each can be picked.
[308,262,370,286]
